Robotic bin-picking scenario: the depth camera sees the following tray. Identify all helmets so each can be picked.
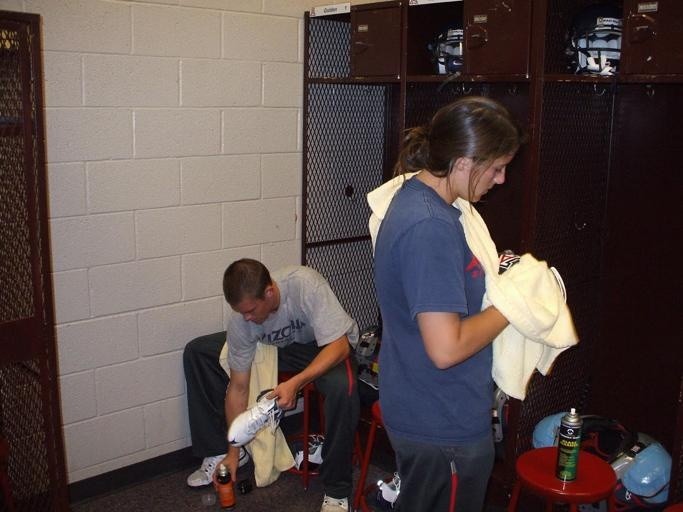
[430,26,464,75]
[574,16,622,75]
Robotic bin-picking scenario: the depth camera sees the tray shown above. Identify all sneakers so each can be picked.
[292,434,325,471]
[377,471,401,507]
[319,495,349,512]
[227,390,282,448]
[186,446,250,488]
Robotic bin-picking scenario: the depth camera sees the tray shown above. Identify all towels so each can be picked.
[366,170,581,402]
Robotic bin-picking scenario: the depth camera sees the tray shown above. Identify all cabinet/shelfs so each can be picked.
[345,0,683,83]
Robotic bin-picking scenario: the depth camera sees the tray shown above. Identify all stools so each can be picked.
[276,371,361,492]
[507,446,618,511]
[352,398,457,511]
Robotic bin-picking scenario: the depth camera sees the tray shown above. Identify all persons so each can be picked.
[182,257,362,512]
[373,91,522,510]
